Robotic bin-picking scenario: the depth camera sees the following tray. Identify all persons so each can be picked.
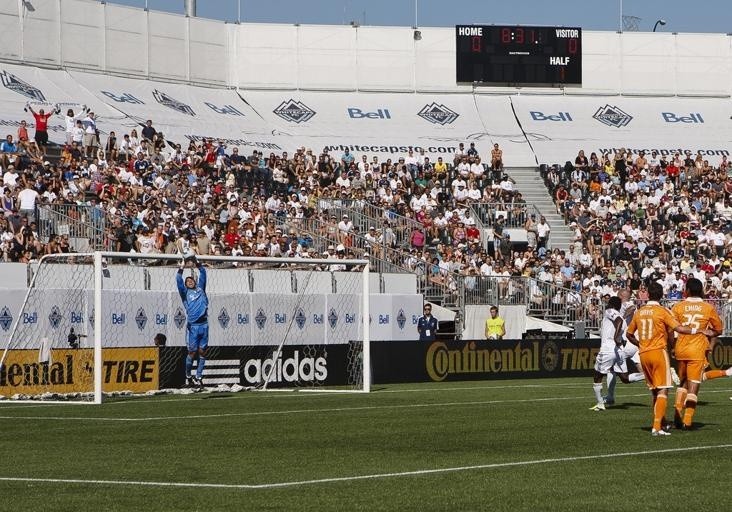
[484,306,506,340]
[668,278,723,431]
[703,366,732,382]
[1,101,377,272]
[601,288,643,403]
[540,147,732,325]
[588,295,681,411]
[365,142,551,302]
[175,252,210,389]
[626,283,706,434]
[417,303,440,340]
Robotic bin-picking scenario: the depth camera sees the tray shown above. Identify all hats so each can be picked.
[322,245,334,255]
[342,214,348,218]
[44,161,50,166]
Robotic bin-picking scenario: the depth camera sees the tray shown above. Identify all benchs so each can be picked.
[417,276,601,337]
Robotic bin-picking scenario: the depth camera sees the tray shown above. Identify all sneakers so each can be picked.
[652,425,672,437]
[589,395,614,411]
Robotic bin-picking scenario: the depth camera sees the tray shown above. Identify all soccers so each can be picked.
[183,248,195,259]
